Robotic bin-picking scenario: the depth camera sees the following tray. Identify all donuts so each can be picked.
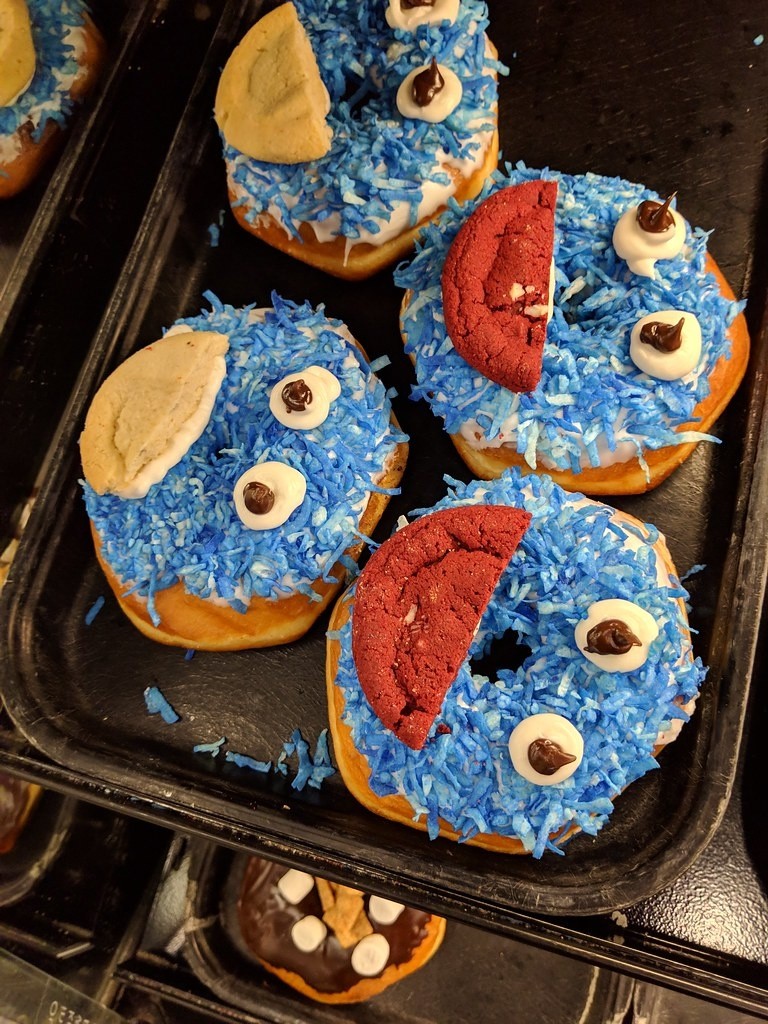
[325,474,700,857]
[1,1,102,194]
[211,0,511,281]
[222,850,444,1005]
[398,155,750,497]
[78,301,409,651]
